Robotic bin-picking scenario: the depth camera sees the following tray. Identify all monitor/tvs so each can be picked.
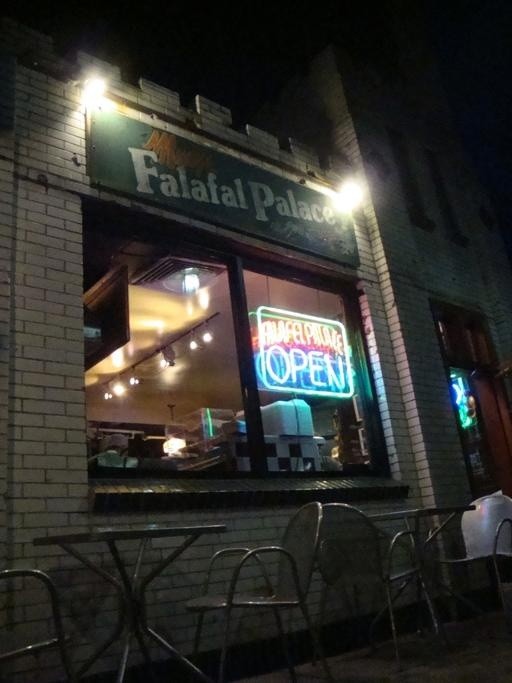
[83,263,134,370]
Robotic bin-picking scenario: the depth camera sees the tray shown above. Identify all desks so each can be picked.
[366,504,478,652]
[33,524,228,681]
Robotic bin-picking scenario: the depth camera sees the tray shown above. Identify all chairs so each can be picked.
[185,502,335,681]
[419,494,511,634]
[319,503,439,673]
[2,567,75,683]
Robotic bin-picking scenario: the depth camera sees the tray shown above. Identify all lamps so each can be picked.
[102,311,220,400]
